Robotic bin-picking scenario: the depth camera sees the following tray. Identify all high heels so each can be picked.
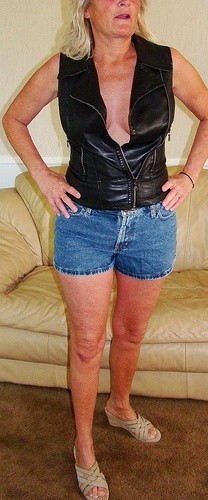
[105,405,161,443]
[73,445,109,500]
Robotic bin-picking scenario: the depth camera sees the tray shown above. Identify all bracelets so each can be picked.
[180,172,194,188]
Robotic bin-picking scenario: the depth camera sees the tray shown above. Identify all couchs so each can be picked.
[0,164,208,402]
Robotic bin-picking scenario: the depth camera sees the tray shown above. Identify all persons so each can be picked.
[3,0,208,500]
